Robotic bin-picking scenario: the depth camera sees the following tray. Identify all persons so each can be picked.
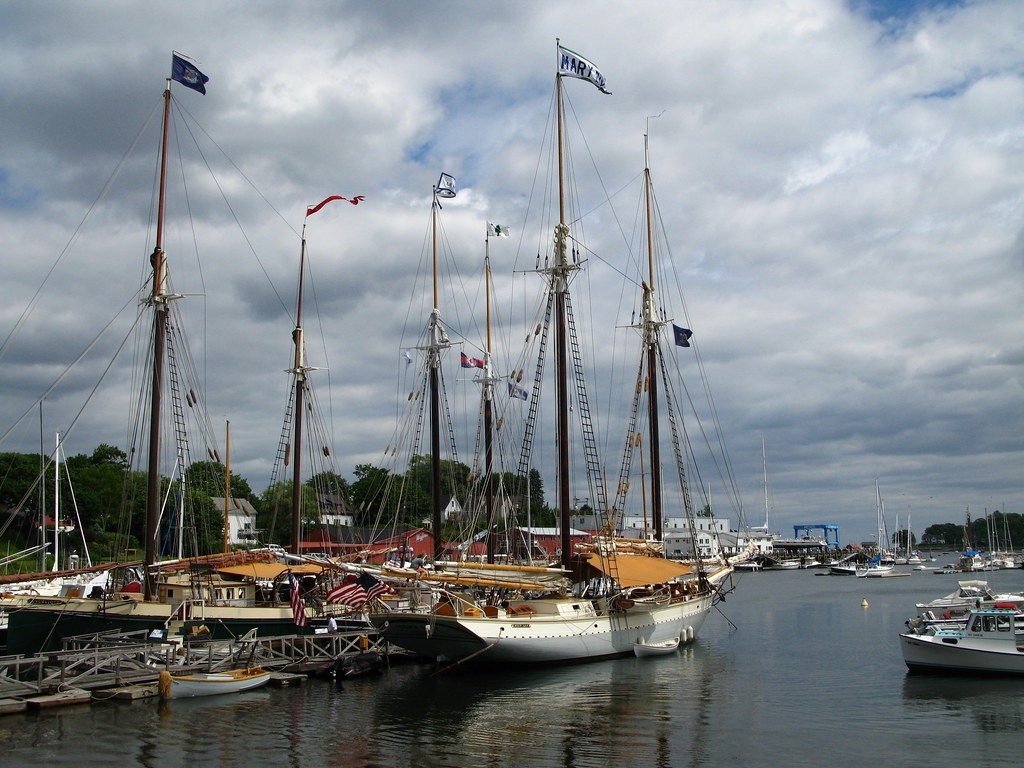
[412,553,428,578]
[326,613,338,654]
[647,581,681,595]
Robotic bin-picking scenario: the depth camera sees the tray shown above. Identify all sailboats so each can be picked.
[0,37,1024,716]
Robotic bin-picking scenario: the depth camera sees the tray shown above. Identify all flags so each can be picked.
[558,45,612,95]
[289,572,307,626]
[435,172,456,198]
[487,222,510,236]
[673,324,692,347]
[509,382,529,401]
[172,54,209,95]
[326,572,396,610]
[461,352,483,369]
[306,195,366,217]
